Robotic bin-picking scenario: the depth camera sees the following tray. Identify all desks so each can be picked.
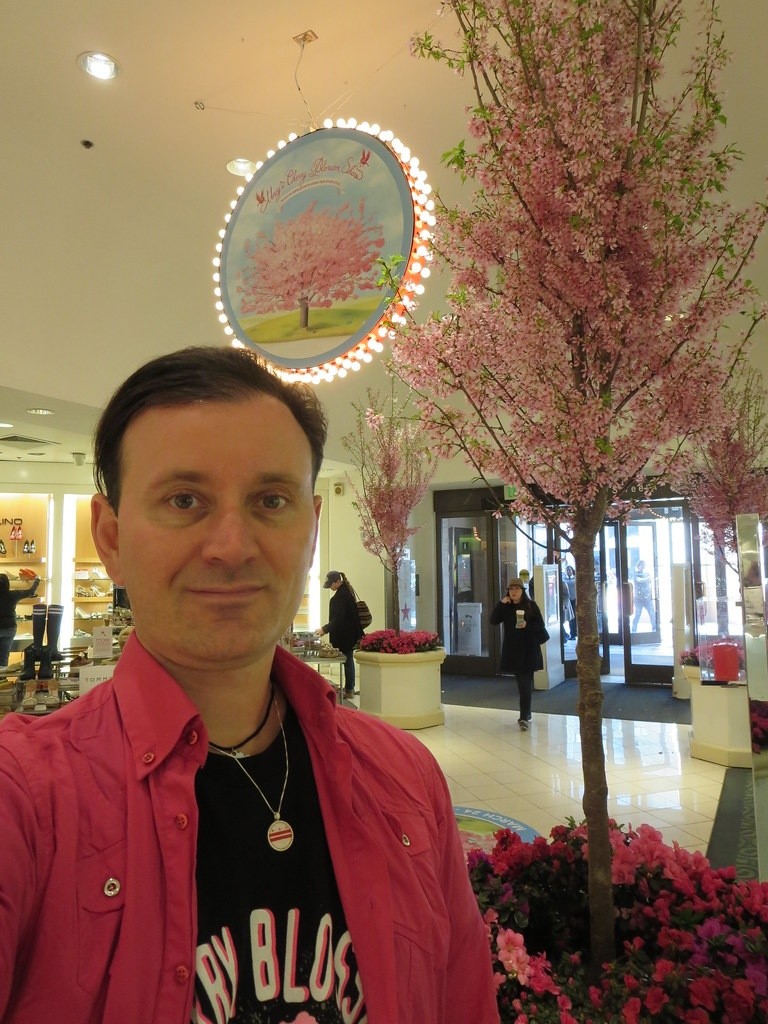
[295,650,347,705]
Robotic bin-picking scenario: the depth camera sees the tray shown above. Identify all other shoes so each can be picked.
[0,525,37,580]
[36,680,42,692]
[71,656,86,667]
[42,681,47,691]
[520,720,528,731]
[0,662,23,692]
[342,688,353,699]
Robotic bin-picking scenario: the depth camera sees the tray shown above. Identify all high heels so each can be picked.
[75,567,114,639]
[22,679,37,710]
[45,679,59,708]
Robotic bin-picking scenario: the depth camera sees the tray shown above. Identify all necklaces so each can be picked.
[208,682,296,853]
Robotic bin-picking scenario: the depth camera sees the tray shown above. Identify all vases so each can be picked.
[682,665,701,681]
[353,646,447,729]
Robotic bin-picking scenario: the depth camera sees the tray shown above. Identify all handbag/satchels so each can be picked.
[353,589,372,629]
[528,600,549,644]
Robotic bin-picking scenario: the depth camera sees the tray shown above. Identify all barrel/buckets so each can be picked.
[714,644,739,682]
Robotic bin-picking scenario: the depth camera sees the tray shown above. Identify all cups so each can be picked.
[516,609,525,628]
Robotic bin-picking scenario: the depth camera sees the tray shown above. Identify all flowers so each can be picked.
[679,638,745,668]
[341,377,443,654]
[749,697,768,754]
[368,0,768,1024]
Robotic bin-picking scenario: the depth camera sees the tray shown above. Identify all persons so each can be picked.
[529,556,608,645]
[490,578,549,730]
[0,350,498,1024]
[314,571,366,699]
[0,573,41,666]
[631,560,656,633]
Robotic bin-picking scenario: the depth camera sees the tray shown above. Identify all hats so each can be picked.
[506,578,527,589]
[323,571,340,588]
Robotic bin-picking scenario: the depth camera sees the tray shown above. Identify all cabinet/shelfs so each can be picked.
[0,557,51,652]
[72,557,113,639]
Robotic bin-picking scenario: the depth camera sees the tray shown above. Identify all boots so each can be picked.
[33,604,47,658]
[19,647,36,680]
[38,646,53,678]
[46,606,63,660]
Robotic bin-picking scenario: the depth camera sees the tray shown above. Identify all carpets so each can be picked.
[440,673,690,725]
[326,679,359,709]
